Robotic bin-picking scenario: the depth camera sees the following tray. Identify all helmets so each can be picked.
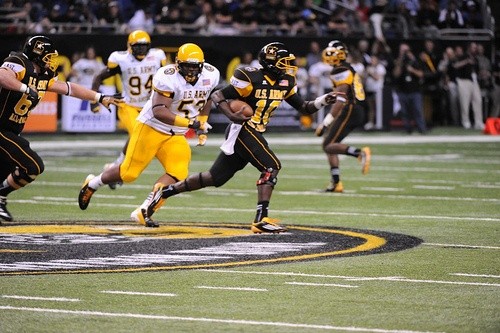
[258,43,299,77]
[127,29,152,61]
[322,40,349,64]
[23,36,59,70]
[175,43,204,83]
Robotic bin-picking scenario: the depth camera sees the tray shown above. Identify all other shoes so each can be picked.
[359,146,373,174]
[321,181,344,193]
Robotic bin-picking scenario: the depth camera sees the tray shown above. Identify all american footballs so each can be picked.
[226,100,254,117]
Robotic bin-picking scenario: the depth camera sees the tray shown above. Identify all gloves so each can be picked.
[25,88,40,101]
[196,129,208,148]
[100,92,125,113]
[188,120,212,133]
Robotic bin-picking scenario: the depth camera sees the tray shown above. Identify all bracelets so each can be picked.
[19,83,27,93]
[95,93,102,101]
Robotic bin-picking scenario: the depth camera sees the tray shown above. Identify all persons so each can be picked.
[152,41,346,234]
[0,0,500,190]
[79,42,220,227]
[0,36,128,219]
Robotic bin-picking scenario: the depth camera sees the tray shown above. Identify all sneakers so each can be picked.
[0,195,14,221]
[130,211,160,227]
[145,184,166,218]
[250,217,288,233]
[77,173,98,211]
